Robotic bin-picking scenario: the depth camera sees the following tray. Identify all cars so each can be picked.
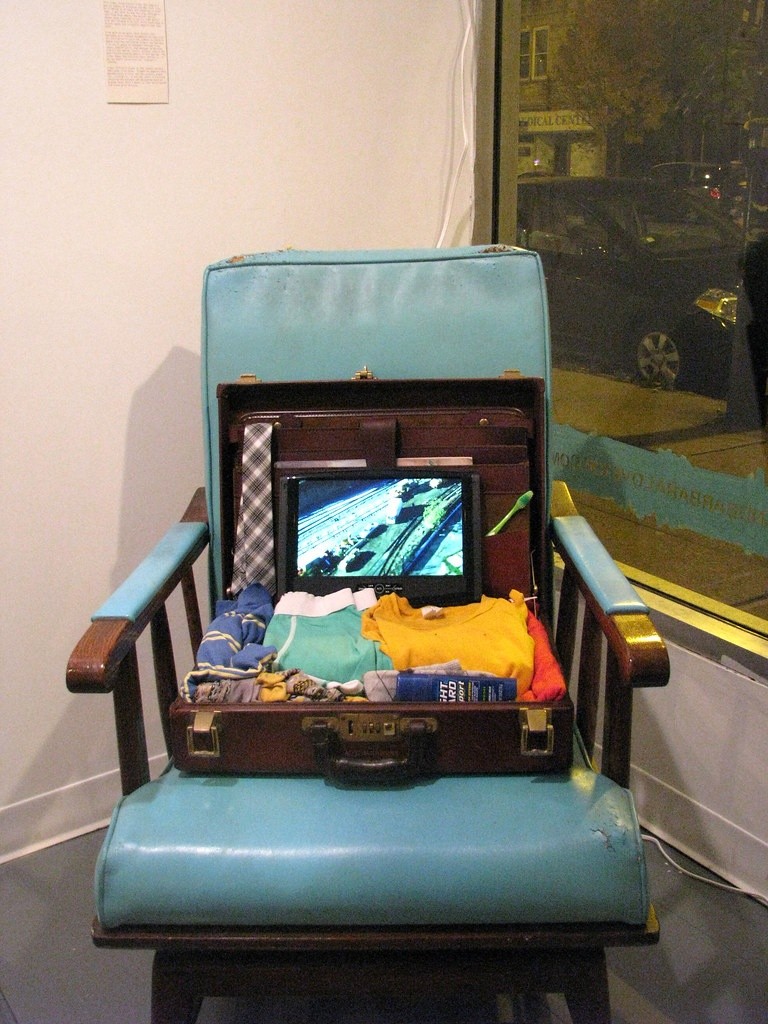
[518,175,767,398]
[646,161,738,217]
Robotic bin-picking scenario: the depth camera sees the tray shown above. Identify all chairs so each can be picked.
[63,242,675,1024]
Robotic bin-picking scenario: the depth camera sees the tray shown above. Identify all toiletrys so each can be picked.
[395,672,517,703]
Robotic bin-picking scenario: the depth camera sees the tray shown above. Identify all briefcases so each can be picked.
[168,370,576,787]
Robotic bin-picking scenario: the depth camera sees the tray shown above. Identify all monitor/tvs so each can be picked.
[277,468,482,609]
